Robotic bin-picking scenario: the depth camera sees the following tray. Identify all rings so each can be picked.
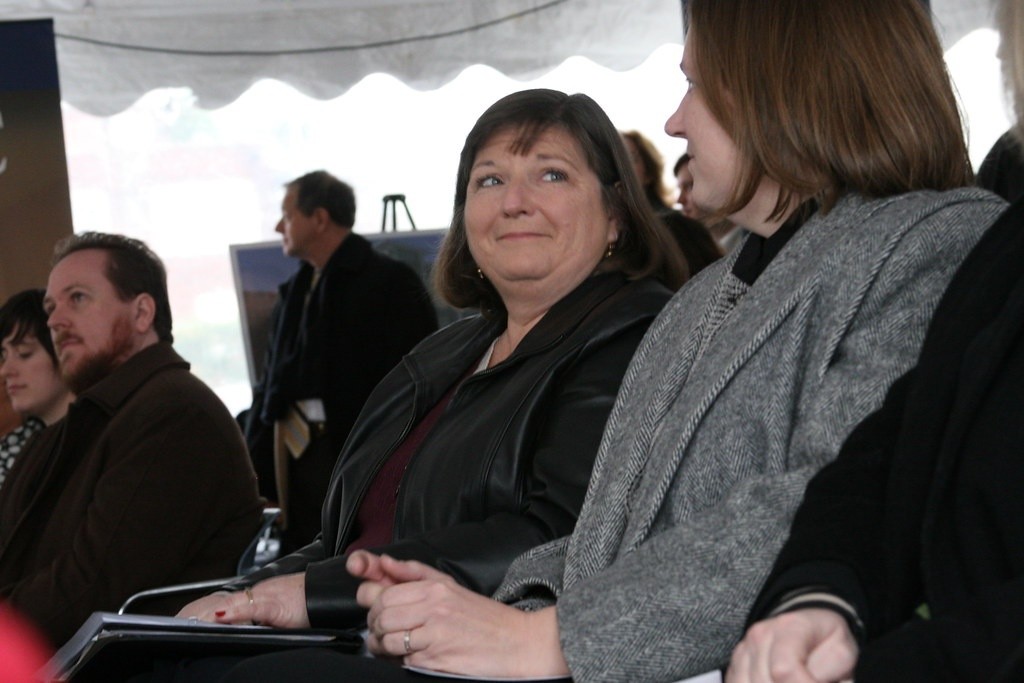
[404,630,414,654]
[244,589,254,604]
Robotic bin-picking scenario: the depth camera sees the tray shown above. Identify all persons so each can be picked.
[243,171,440,549]
[672,153,751,255]
[177,88,689,635]
[726,196,1024,683]
[220,0,1009,683]
[618,130,723,298]
[0,232,265,654]
